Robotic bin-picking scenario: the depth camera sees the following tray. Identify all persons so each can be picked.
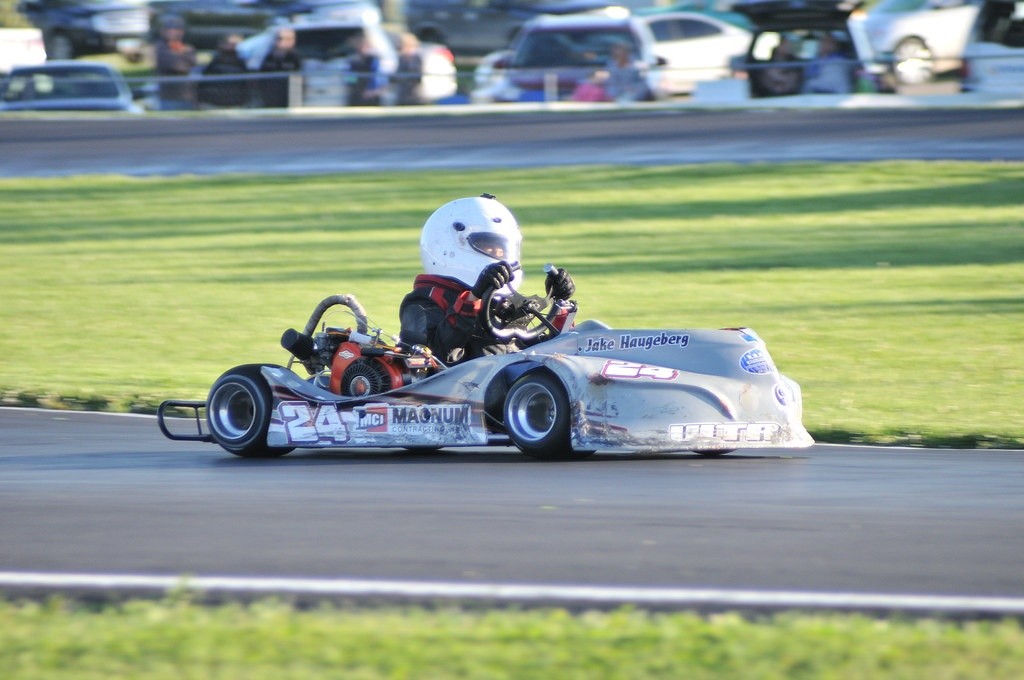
[258,28,301,107]
[399,194,577,368]
[157,14,193,109]
[758,29,857,93]
[570,41,650,101]
[350,22,458,106]
[198,34,249,108]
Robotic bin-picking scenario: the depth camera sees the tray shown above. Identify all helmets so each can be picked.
[420,197,523,302]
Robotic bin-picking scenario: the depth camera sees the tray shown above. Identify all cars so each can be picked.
[234,5,458,114]
[468,1,1024,103]
[0,63,131,112]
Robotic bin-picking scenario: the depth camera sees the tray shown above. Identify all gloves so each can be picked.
[545,268,576,300]
[471,260,514,299]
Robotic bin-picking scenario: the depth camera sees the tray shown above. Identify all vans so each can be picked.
[488,9,675,100]
